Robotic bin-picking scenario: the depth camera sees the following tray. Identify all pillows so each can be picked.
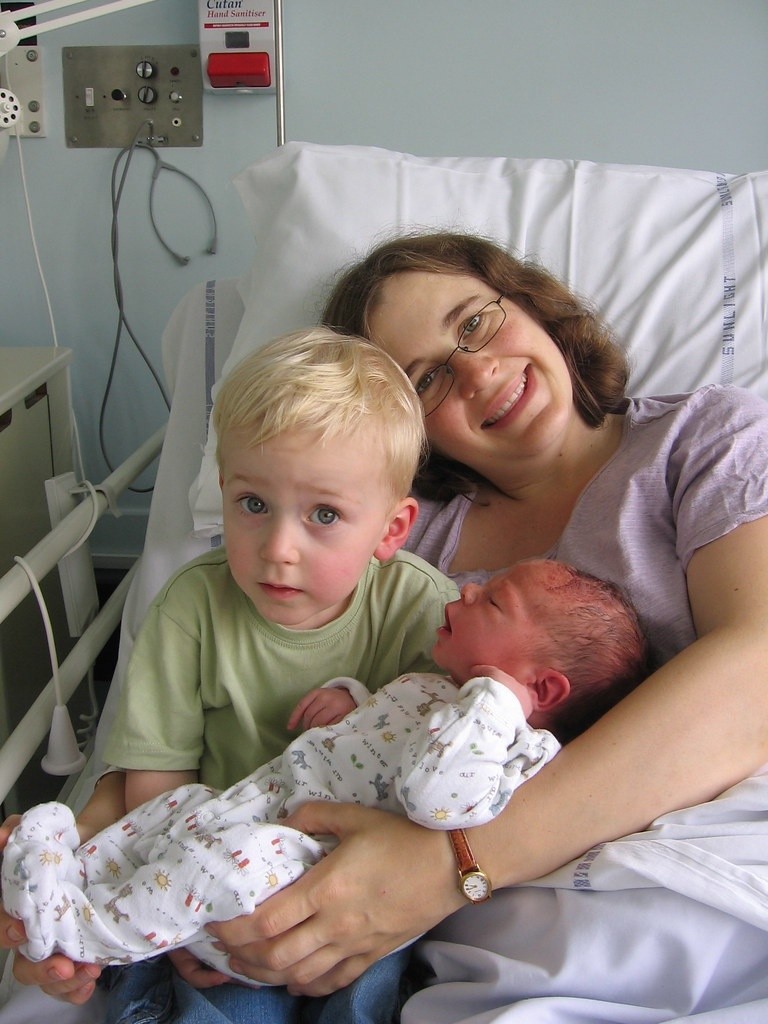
[188,141,768,536]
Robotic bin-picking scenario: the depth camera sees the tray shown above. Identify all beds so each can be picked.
[0,277,250,1024]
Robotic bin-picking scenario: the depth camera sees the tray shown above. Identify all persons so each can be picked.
[0,559,663,987]
[101,324,462,1024]
[0,227,768,1024]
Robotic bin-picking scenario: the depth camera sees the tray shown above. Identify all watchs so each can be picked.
[446,828,493,905]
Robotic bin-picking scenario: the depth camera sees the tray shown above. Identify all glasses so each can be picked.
[417,292,507,417]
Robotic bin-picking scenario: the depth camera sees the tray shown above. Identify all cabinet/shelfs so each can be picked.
[0,346,92,819]
